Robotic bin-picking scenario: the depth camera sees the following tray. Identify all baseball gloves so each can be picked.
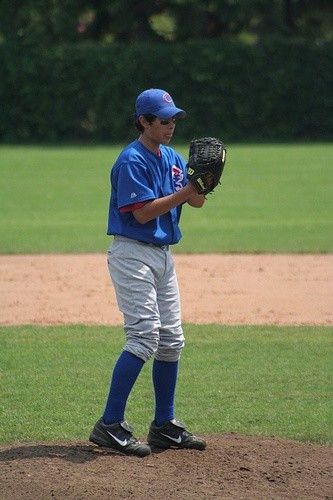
[186,137,226,194]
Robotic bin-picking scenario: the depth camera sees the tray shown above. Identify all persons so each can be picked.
[88,88,207,456]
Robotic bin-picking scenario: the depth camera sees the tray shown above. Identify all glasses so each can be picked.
[160,117,176,125]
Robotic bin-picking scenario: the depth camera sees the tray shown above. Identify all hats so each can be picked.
[135,89,187,119]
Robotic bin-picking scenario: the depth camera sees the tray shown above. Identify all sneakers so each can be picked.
[89,417,151,457]
[147,419,207,450]
